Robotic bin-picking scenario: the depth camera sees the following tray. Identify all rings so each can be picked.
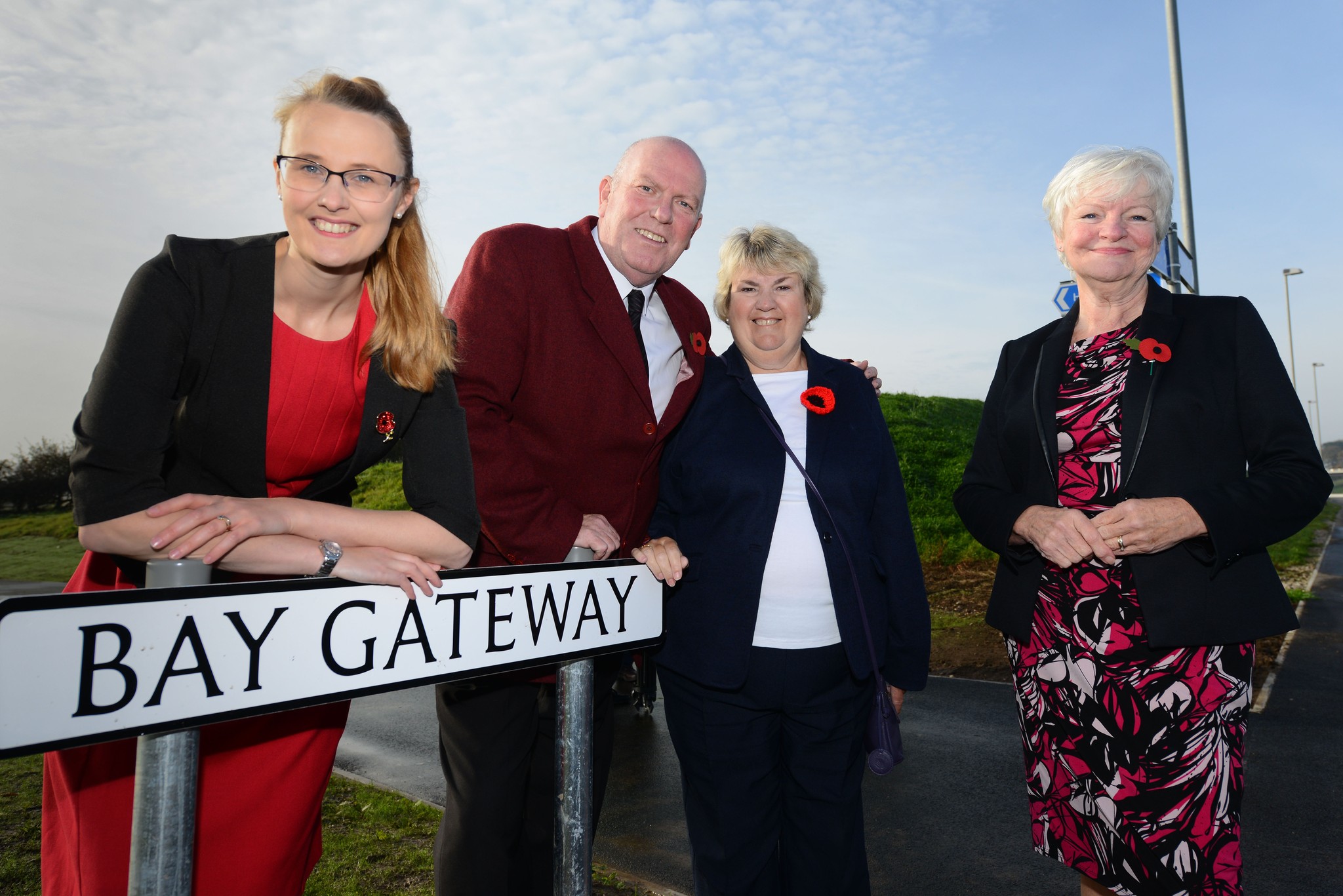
[640,543,652,551]
[218,515,230,532]
[1117,535,1126,551]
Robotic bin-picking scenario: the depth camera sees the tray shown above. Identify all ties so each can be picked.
[627,290,649,382]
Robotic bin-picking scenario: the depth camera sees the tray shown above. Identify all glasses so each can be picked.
[275,155,410,202]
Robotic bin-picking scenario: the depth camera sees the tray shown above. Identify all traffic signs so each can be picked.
[1052,236,1195,318]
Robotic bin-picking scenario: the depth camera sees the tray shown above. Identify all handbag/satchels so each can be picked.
[866,686,904,776]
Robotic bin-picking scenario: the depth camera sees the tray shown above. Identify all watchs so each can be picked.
[305,539,343,579]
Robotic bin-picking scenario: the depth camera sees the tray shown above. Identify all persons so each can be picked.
[37,75,480,896]
[436,137,883,895]
[631,228,930,895]
[953,149,1334,896]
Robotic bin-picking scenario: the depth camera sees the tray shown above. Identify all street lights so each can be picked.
[1284,267,1325,459]
